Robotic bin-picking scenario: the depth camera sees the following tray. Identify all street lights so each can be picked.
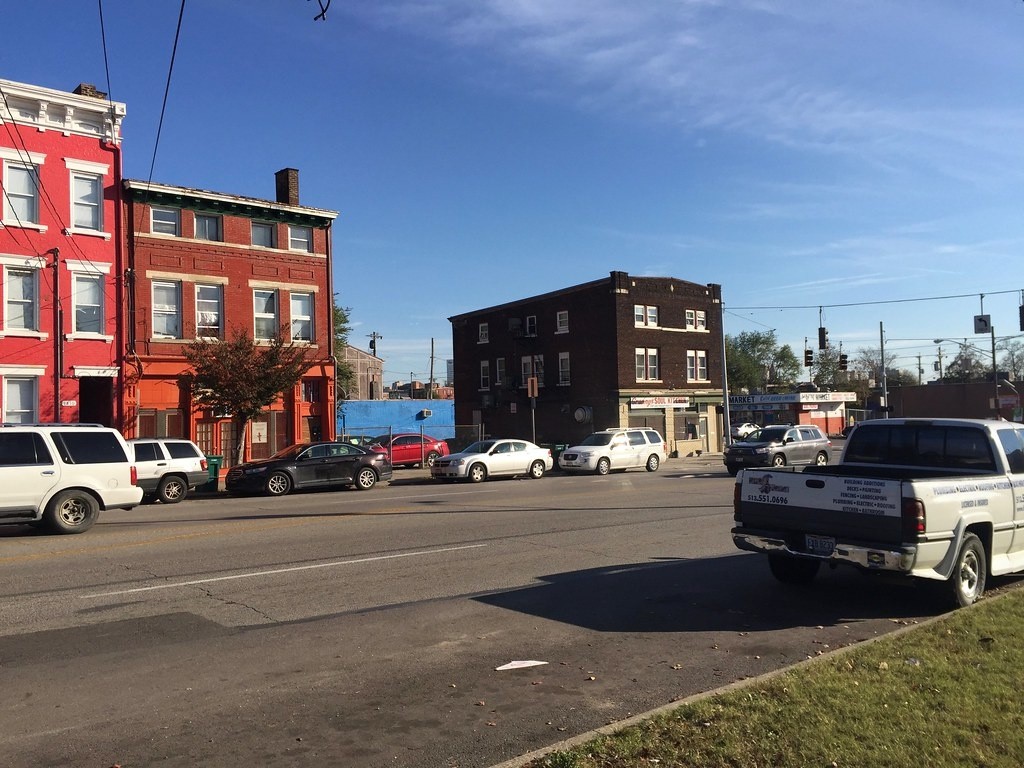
[934,337,1001,419]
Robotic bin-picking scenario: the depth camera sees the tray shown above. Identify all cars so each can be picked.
[119,436,212,505]
[430,439,553,483]
[367,433,450,466]
[225,441,394,497]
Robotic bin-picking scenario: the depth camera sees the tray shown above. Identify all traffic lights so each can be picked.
[840,355,848,370]
[818,327,829,349]
[805,349,814,367]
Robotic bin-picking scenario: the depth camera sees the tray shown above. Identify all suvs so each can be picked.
[724,424,833,474]
[558,426,667,473]
[0,420,144,534]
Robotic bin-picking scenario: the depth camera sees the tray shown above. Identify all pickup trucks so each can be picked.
[731,413,1024,606]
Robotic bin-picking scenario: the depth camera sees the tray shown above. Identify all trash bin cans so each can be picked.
[540,443,569,471]
[195,454,223,492]
